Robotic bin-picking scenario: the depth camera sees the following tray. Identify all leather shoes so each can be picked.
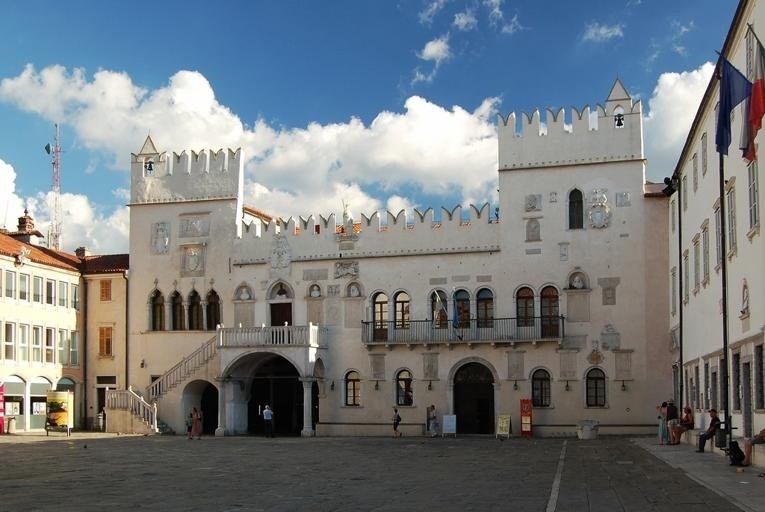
[696,449,704,452]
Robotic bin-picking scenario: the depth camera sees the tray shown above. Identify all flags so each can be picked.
[715,58,752,156]
[740,29,765,162]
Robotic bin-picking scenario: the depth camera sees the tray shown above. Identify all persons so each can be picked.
[185,413,192,438]
[391,408,403,440]
[657,402,669,445]
[675,407,695,443]
[190,406,202,441]
[262,404,274,438]
[428,405,438,438]
[739,427,765,467]
[667,403,680,444]
[695,409,720,453]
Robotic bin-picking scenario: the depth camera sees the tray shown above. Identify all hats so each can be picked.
[708,409,717,413]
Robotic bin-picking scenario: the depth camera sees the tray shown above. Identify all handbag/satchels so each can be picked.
[397,414,401,422]
